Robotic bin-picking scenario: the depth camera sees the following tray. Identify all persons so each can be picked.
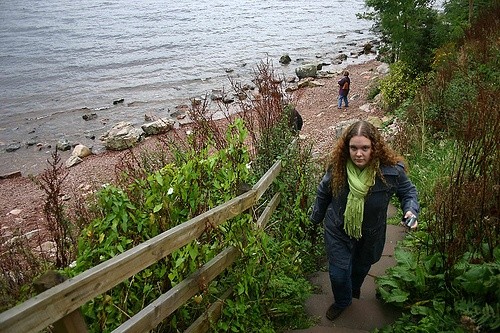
[337,70,351,109]
[290,106,303,133]
[310,120,421,321]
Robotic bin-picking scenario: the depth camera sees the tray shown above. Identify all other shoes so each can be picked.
[326,303,345,321]
[338,107,342,109]
[344,106,348,107]
